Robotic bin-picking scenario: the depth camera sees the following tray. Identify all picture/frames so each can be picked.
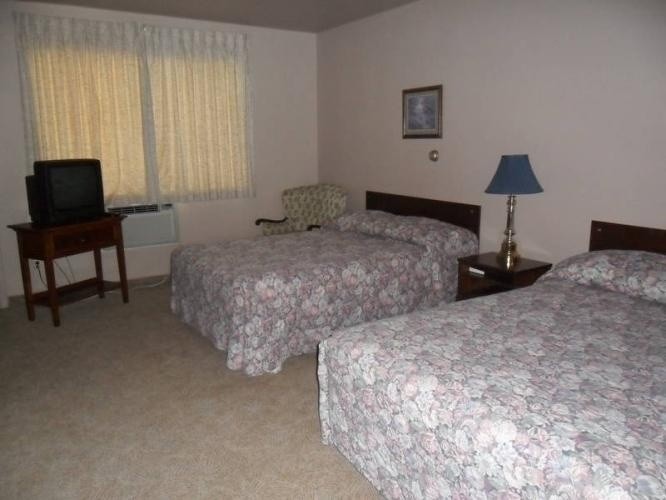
[402,84,443,138]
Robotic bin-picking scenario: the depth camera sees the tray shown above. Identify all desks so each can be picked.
[6,213,129,327]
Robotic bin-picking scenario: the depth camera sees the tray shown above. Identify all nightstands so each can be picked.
[457,252,551,300]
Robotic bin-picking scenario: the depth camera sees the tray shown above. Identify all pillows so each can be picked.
[385,215,479,251]
[322,209,394,235]
[539,251,666,302]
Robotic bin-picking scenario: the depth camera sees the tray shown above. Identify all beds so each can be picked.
[171,190,481,376]
[315,219,666,500]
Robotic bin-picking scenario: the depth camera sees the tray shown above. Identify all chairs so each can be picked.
[255,182,347,235]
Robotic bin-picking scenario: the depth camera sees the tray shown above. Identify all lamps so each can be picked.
[484,155,544,264]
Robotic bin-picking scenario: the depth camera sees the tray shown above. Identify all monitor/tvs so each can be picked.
[25,159,104,228]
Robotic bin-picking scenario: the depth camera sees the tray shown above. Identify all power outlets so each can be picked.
[32,261,42,270]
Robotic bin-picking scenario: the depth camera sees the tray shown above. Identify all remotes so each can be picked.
[104,211,121,217]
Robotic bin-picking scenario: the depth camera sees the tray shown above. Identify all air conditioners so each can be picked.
[100,202,180,250]
